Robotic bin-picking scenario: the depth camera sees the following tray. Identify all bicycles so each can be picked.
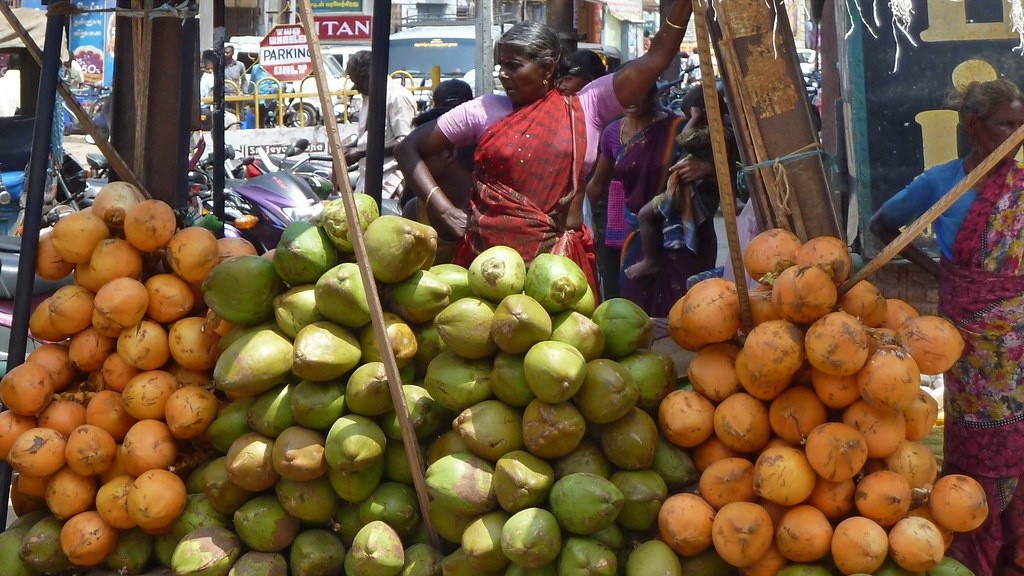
[63,76,112,136]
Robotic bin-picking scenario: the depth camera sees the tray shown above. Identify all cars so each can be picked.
[797,48,815,77]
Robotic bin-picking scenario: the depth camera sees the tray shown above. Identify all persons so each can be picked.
[200,50,214,131]
[584,61,738,317]
[681,47,702,81]
[624,85,738,280]
[559,51,606,96]
[342,50,418,216]
[0,54,20,117]
[63,51,84,89]
[223,46,249,130]
[869,79,1024,576]
[392,0,693,308]
[396,79,476,206]
[401,107,477,268]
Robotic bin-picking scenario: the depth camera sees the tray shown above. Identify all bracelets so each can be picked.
[666,17,687,29]
[426,187,439,205]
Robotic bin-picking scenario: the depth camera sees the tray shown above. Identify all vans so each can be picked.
[223,42,356,126]
[330,46,372,70]
[388,25,622,113]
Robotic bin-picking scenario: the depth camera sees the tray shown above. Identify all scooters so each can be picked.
[0,124,361,363]
[657,50,820,117]
